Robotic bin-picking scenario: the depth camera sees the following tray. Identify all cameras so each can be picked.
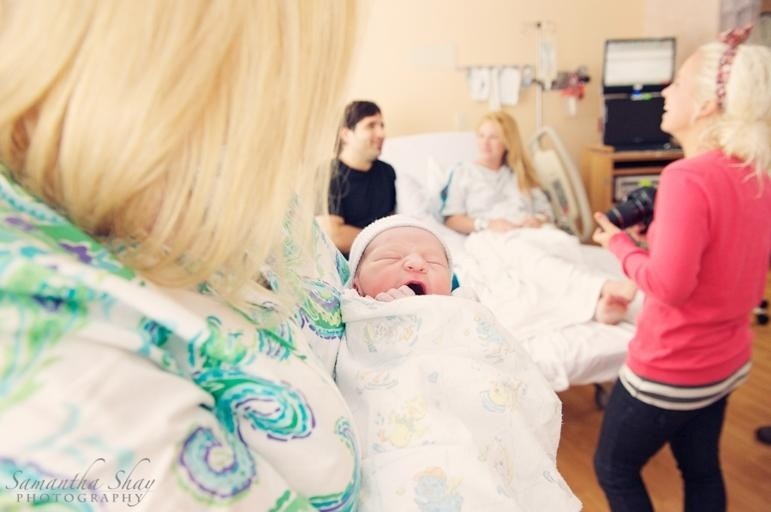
[605,186,657,234]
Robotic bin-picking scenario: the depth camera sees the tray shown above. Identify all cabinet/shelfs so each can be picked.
[568,139,685,243]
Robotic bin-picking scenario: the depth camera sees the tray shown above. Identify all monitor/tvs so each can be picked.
[601,37,675,100]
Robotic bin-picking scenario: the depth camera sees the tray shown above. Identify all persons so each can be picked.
[440,109,555,230]
[313,99,398,261]
[591,27,771,512]
[340,215,456,298]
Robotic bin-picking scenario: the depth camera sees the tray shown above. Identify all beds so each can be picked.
[382,118,646,416]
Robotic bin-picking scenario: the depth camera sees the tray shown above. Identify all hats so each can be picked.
[344,214,454,288]
